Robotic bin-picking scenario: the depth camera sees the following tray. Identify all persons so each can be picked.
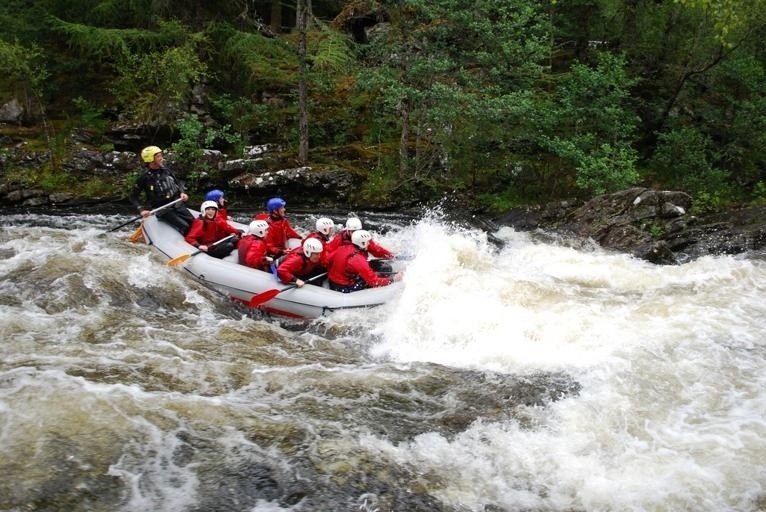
[128,145,195,238]
[184,189,404,294]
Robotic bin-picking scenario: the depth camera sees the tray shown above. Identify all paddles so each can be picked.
[167,235,234,267]
[249,271,329,307]
[368,249,415,263]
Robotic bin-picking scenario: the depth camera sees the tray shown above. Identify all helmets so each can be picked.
[316,218,334,235]
[249,220,269,238]
[141,146,162,163]
[267,198,286,211]
[346,218,372,249]
[303,237,323,257]
[200,190,224,217]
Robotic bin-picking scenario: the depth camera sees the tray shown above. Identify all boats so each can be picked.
[140,207,404,322]
